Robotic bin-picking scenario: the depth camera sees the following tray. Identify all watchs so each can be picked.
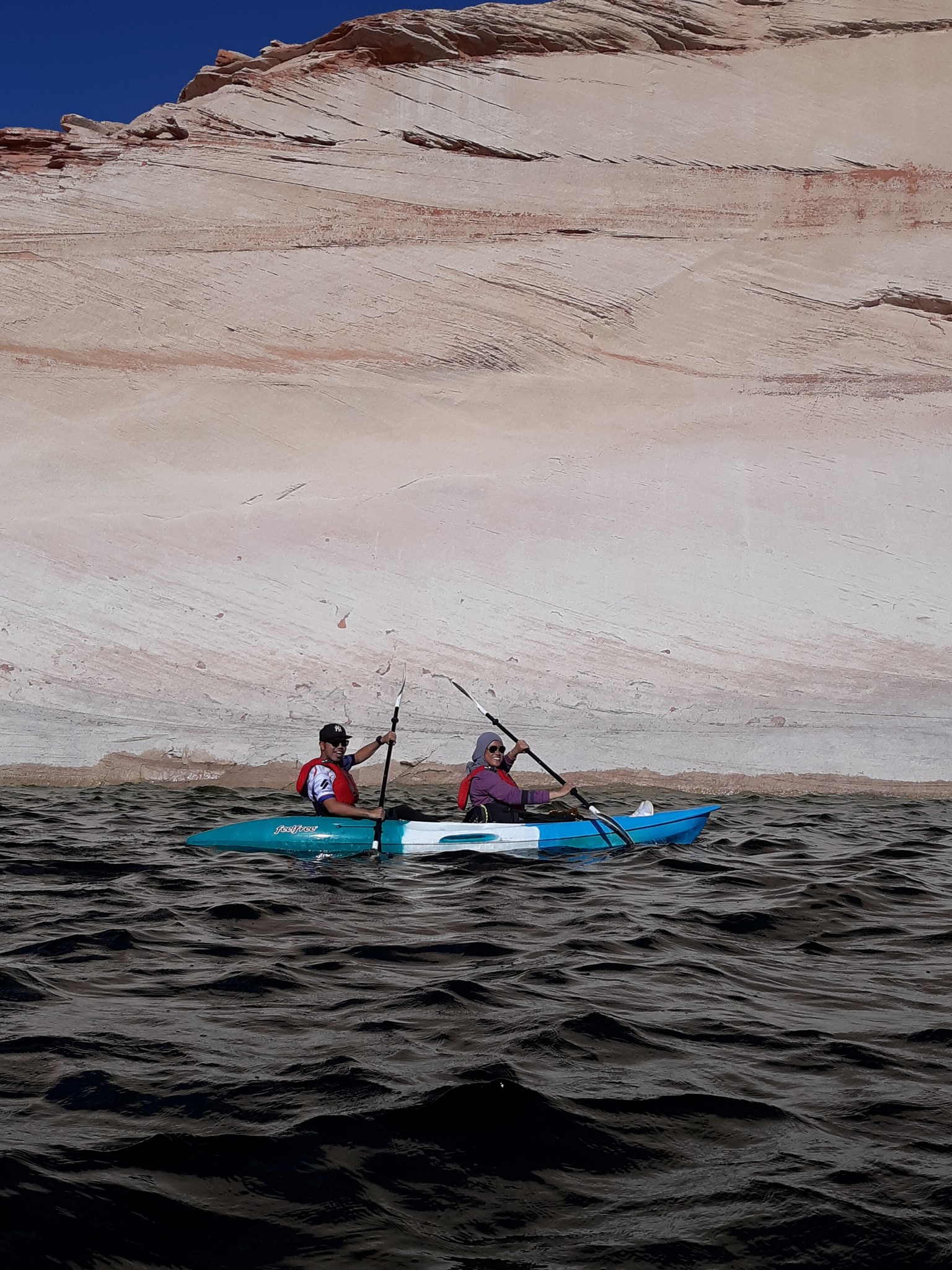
[376,735,385,745]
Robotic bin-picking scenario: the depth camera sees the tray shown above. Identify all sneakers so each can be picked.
[473,804,488,823]
[463,806,477,823]
[628,802,645,818]
[636,800,654,817]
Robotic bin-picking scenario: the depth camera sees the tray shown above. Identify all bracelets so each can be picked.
[510,751,517,759]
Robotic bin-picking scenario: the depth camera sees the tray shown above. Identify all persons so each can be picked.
[307,723,439,822]
[465,732,654,822]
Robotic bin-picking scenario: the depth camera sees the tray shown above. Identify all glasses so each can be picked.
[326,740,349,747]
[486,746,506,753]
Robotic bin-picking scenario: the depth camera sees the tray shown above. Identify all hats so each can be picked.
[319,723,353,741]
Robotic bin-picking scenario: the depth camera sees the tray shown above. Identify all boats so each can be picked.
[186,804,721,856]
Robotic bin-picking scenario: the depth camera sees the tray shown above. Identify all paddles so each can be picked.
[450,680,636,852]
[369,662,408,855]
